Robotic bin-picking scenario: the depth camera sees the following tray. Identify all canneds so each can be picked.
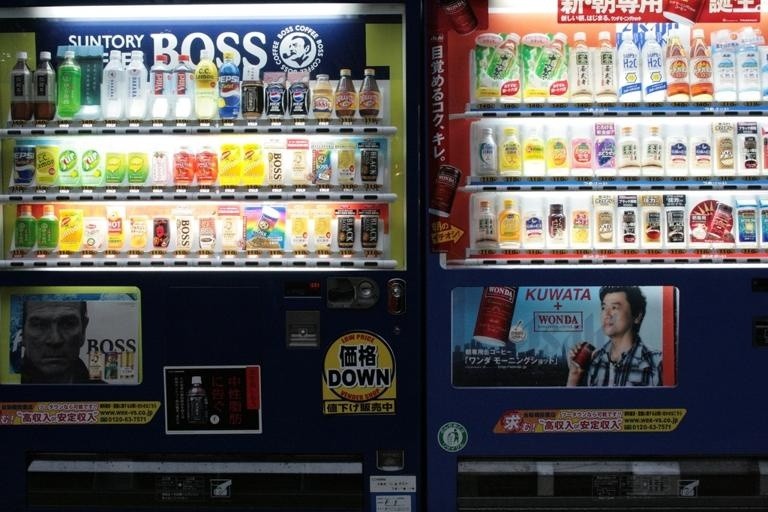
[242,82,264,116]
[35,145,60,185]
[286,82,311,114]
[706,201,734,237]
[13,146,37,184]
[265,83,287,116]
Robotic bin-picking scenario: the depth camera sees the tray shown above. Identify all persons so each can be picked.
[565,287,663,386]
[19,302,107,385]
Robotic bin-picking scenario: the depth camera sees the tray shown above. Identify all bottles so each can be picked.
[186,376,208,425]
[478,116,768,179]
[13,131,380,189]
[11,49,382,126]
[438,0,479,37]
[662,0,707,25]
[474,27,768,104]
[472,189,768,251]
[14,199,382,253]
[89,350,136,381]
